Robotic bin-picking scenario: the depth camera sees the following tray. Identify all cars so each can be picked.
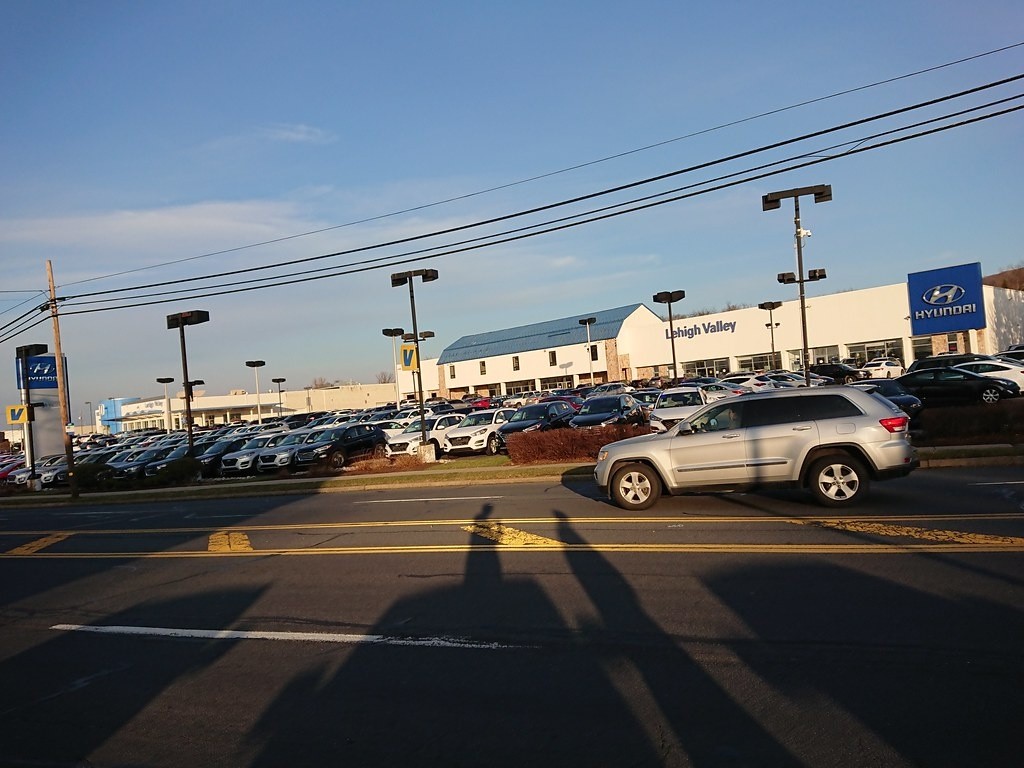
[0,344,1023,489]
[594,384,921,511]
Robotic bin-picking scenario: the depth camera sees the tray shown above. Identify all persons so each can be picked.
[699,404,739,433]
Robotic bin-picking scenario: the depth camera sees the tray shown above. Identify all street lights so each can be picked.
[762,184,832,386]
[391,268,439,465]
[653,290,686,388]
[167,310,210,458]
[579,317,596,386]
[759,300,782,368]
[382,328,405,411]
